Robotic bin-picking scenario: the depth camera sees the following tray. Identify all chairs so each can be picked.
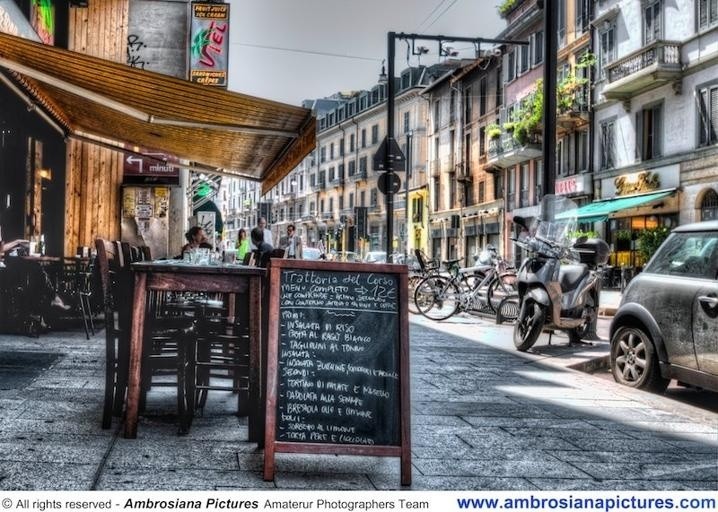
[93,234,291,446]
[11,253,91,339]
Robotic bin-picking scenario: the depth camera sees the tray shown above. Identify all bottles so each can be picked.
[27,234,45,256]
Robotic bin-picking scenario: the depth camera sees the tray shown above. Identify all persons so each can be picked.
[182,217,303,265]
[0,227,72,329]
[316,233,328,261]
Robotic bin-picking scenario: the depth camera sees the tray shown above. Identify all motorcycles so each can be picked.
[509,194,609,352]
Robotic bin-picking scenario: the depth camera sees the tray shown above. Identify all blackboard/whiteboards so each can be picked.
[268,257,412,456]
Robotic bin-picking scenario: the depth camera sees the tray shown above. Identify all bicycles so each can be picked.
[390,248,518,321]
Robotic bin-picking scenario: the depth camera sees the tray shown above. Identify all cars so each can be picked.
[608,221,717,393]
[337,251,388,263]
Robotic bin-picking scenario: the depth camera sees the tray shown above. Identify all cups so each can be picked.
[181,247,223,266]
